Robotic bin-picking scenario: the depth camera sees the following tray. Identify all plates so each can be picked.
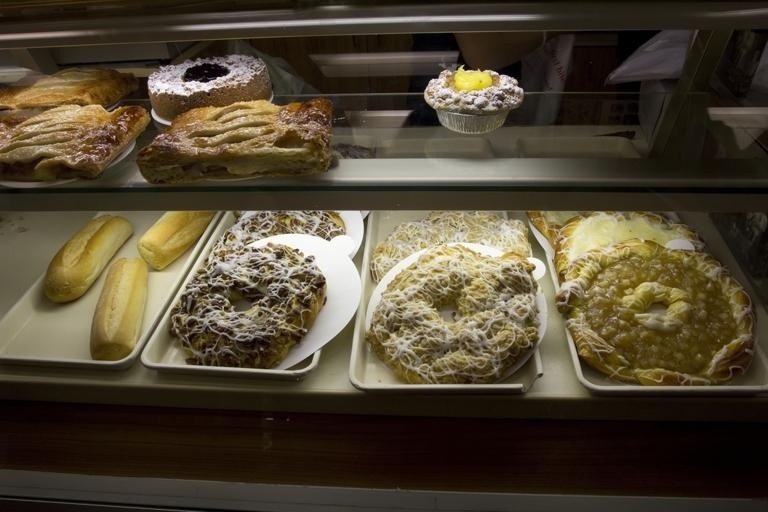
[149,89,275,126]
[205,172,259,184]
[360,210,369,220]
[104,101,120,116]
[240,210,365,258]
[245,232,359,371]
[364,242,547,385]
[4,136,137,190]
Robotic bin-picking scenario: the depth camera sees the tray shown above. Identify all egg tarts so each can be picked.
[423,65,525,137]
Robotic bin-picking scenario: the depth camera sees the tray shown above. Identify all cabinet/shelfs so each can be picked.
[0,0,768,510]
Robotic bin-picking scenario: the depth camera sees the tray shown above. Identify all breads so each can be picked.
[0,66,153,187]
[135,52,337,185]
[90,257,148,361]
[136,210,217,272]
[42,214,135,303]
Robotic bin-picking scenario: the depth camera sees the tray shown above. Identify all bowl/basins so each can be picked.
[438,107,509,134]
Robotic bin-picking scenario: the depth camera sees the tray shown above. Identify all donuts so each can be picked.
[358,210,543,390]
[165,210,349,371]
[523,205,757,393]
